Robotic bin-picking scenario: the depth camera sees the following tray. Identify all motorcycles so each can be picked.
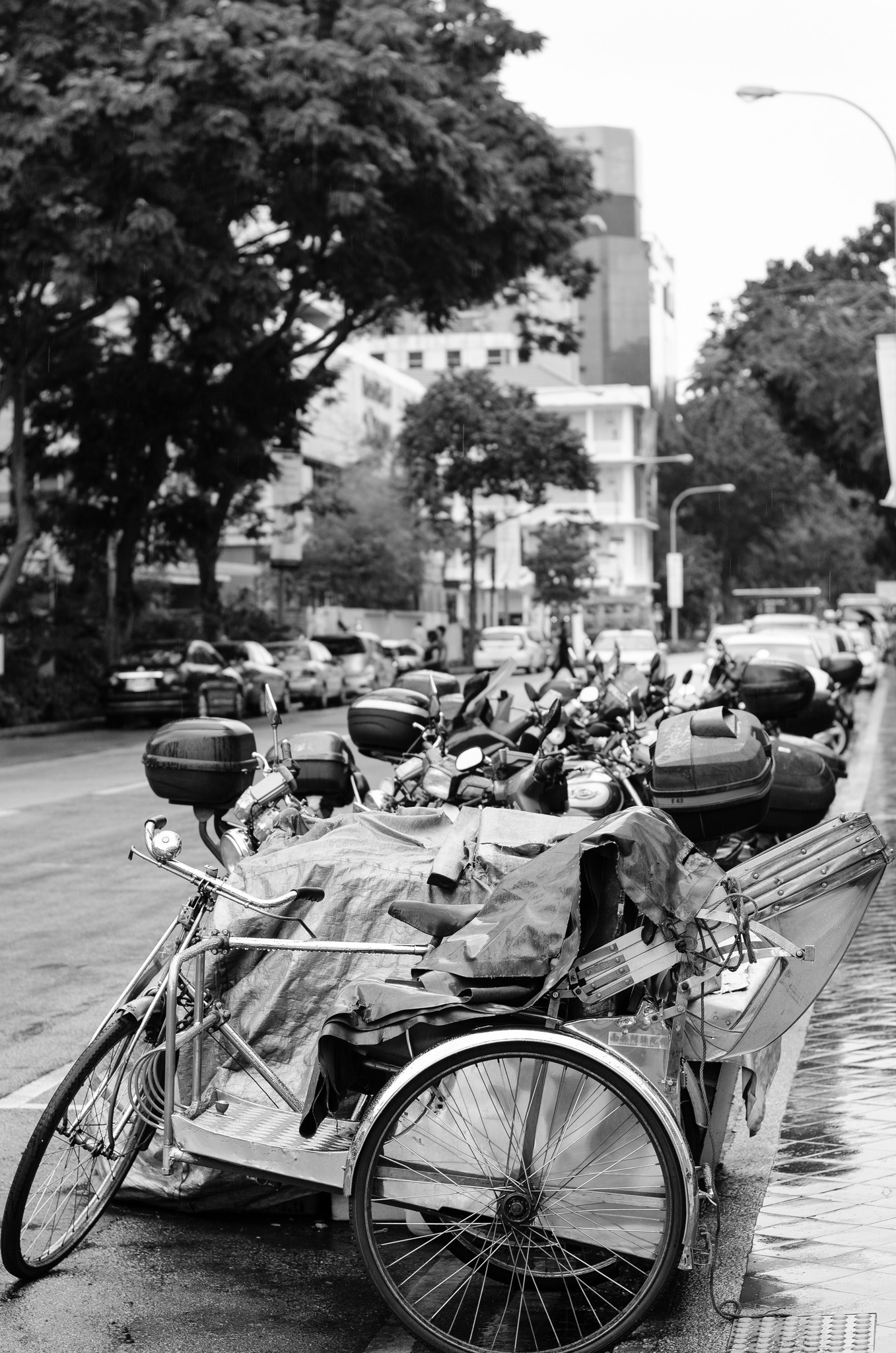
[138,642,861,1027]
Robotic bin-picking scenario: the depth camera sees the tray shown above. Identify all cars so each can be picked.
[470,626,668,686]
[698,594,892,692]
[101,630,425,725]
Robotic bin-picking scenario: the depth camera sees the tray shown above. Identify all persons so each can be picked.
[412,620,446,672]
[551,632,577,678]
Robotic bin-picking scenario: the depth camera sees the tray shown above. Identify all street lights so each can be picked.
[670,484,737,653]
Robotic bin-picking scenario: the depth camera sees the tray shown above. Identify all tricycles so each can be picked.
[2,816,695,1352]
